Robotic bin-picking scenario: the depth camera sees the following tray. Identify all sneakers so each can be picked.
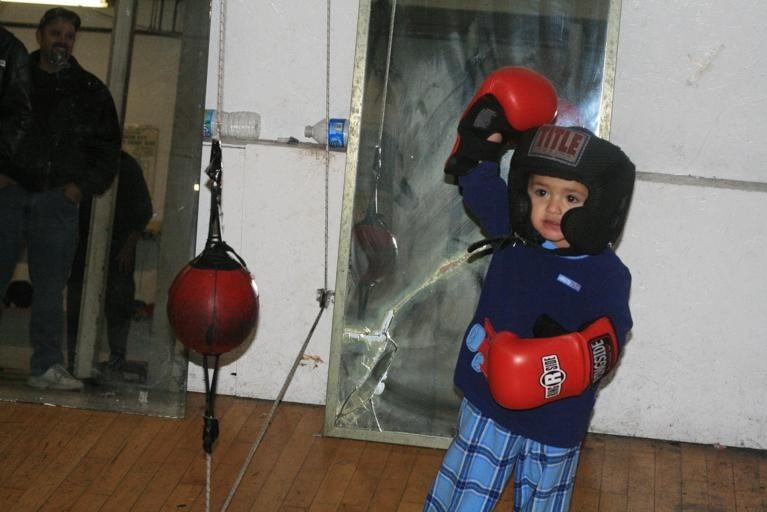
[108,355,126,371]
[28,364,84,390]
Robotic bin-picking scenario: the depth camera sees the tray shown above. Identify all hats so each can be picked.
[508,125,635,256]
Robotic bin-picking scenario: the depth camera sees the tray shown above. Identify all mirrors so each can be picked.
[322,1,622,451]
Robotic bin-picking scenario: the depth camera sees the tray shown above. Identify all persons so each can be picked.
[67,148,153,373]
[2,8,121,392]
[423,67,636,512]
[1,28,32,188]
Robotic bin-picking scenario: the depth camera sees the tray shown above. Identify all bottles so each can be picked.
[304,118,348,147]
[202,107,261,141]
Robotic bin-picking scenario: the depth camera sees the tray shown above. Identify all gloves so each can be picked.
[488,315,618,409]
[445,67,557,185]
